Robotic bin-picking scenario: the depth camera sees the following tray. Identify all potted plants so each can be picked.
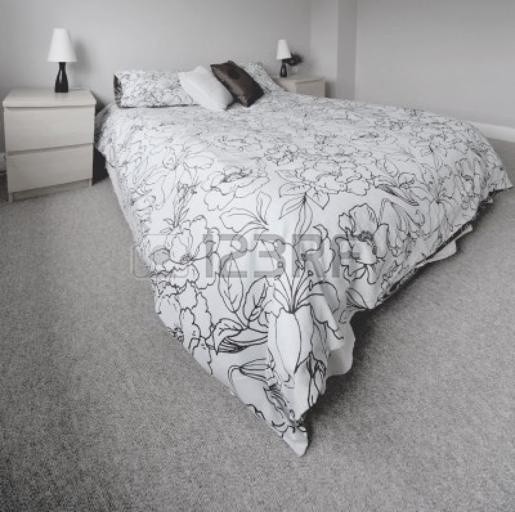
[286,53,304,73]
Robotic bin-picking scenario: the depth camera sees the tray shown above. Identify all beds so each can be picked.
[95,92,513,459]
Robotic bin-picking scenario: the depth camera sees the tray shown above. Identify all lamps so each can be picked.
[276,40,292,77]
[47,28,78,92]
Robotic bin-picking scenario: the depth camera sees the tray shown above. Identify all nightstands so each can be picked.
[2,88,97,202]
[272,74,325,97]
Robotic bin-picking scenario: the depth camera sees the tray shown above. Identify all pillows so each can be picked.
[114,60,284,113]
